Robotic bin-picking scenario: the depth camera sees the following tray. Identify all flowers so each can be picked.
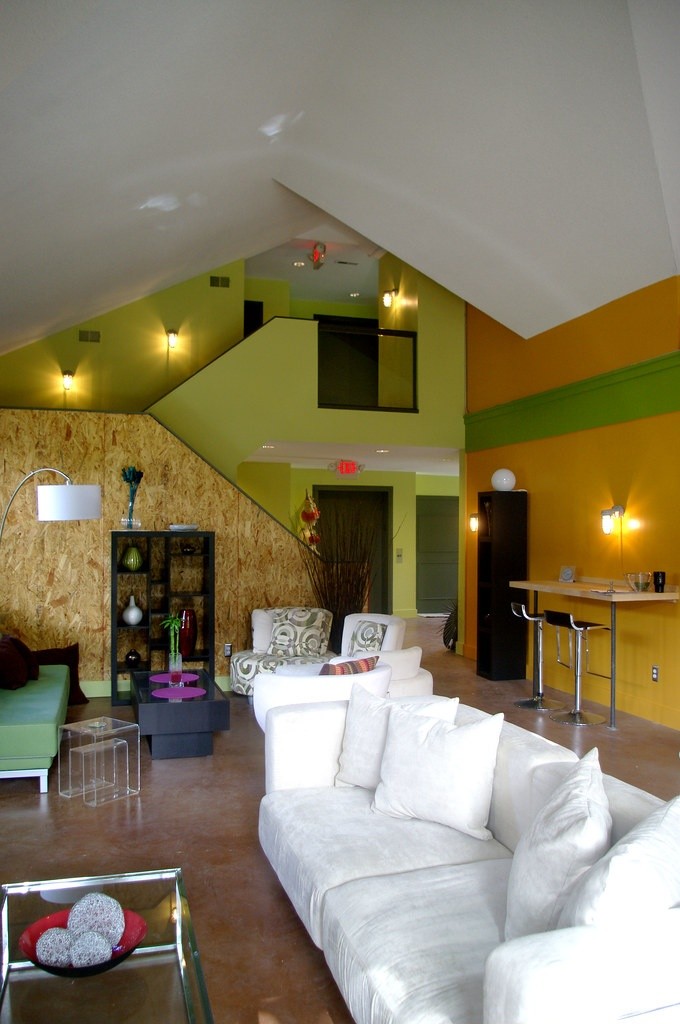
[121,466,144,528]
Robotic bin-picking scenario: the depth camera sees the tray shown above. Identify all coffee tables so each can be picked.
[0,867,214,1024]
[130,669,230,759]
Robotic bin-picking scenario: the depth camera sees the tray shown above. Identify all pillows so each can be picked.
[557,796,679,927]
[372,705,504,842]
[266,608,325,657]
[9,635,39,679]
[0,635,29,690]
[319,656,380,675]
[32,642,89,706]
[348,620,388,656]
[334,684,460,792]
[503,747,612,942]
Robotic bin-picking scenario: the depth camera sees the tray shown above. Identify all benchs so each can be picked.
[0,665,70,793]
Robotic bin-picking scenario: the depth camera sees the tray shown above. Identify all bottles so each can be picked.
[177,608,197,659]
[123,595,143,626]
[126,649,141,668]
[123,547,143,572]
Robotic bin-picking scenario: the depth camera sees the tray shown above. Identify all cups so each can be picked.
[653,571,665,593]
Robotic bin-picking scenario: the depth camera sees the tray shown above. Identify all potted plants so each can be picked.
[160,612,183,688]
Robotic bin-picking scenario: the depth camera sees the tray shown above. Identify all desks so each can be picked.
[510,579,680,731]
[58,716,140,807]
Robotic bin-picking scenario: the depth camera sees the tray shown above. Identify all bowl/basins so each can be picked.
[18,908,148,979]
[624,572,652,593]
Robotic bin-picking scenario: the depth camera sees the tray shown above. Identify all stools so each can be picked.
[543,609,612,725]
[511,603,569,712]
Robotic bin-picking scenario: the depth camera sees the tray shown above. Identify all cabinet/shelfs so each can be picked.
[110,530,215,706]
[476,491,528,681]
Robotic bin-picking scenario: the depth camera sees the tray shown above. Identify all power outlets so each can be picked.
[652,666,659,682]
[224,644,232,657]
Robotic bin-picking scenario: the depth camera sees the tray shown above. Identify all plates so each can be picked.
[170,524,199,532]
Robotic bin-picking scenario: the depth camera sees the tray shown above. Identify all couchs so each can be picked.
[257,694,680,1024]
[230,606,433,734]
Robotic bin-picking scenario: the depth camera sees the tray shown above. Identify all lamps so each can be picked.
[600,505,624,535]
[166,329,178,348]
[329,459,365,480]
[0,468,102,546]
[382,288,399,308]
[308,243,325,269]
[470,512,478,532]
[63,369,74,390]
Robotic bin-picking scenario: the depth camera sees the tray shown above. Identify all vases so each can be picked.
[122,596,142,625]
[121,477,143,529]
[179,609,197,658]
[123,548,143,572]
[126,649,141,668]
